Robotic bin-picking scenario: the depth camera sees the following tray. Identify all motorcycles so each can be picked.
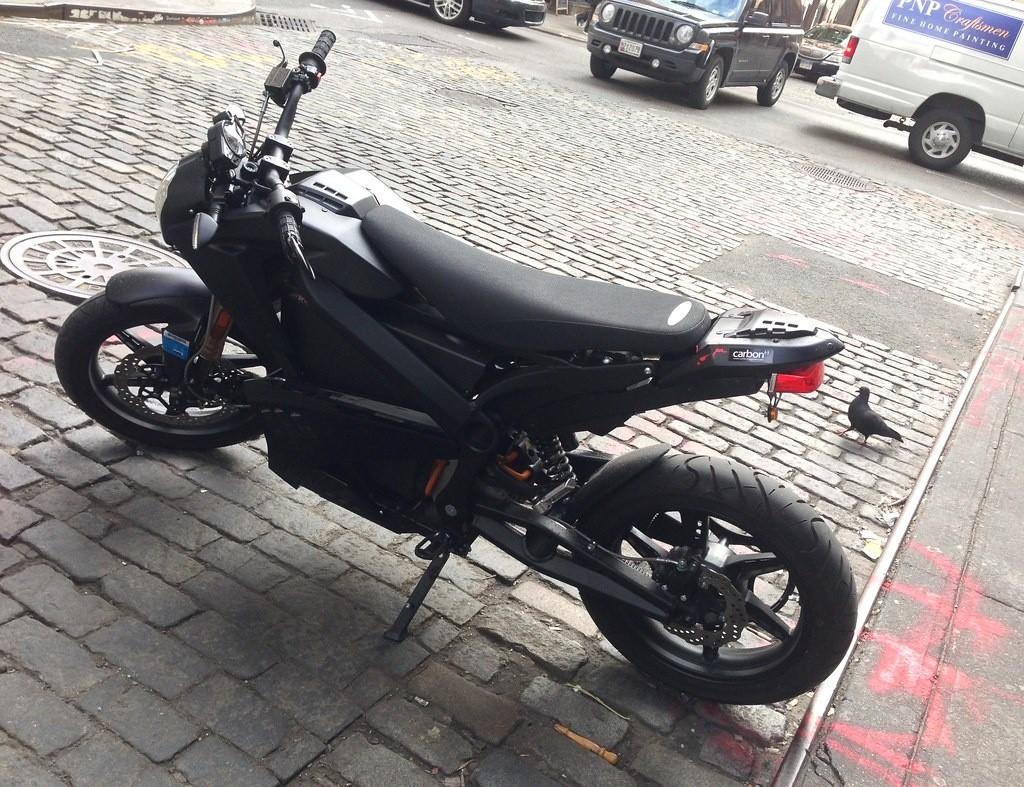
[52,28,859,706]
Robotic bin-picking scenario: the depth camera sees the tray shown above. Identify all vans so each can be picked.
[812,0,1024,172]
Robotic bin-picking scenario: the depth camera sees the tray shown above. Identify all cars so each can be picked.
[389,1,548,29]
[795,22,851,82]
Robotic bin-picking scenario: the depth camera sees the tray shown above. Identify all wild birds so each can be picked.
[834,386,903,447]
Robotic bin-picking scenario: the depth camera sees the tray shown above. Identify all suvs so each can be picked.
[587,1,805,110]
